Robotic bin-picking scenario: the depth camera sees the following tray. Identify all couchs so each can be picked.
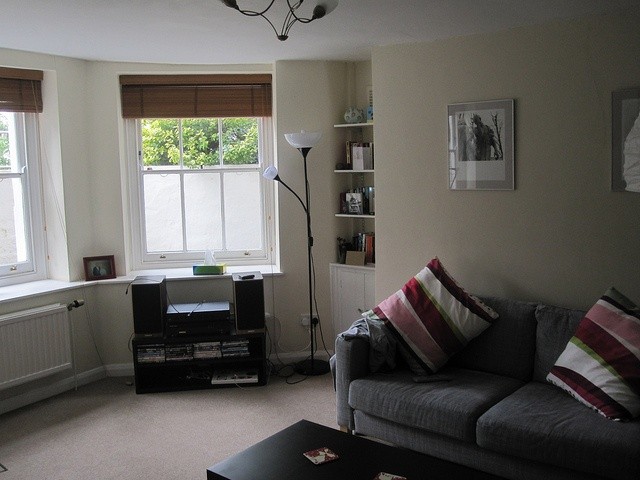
[332,294,640,480]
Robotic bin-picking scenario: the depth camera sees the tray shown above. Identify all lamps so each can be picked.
[219,0,340,41]
[262,130,331,376]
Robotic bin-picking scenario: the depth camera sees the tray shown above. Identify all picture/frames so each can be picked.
[444,98,517,192]
[608,88,640,193]
[82,255,116,282]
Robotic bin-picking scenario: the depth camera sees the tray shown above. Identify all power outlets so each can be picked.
[299,313,318,327]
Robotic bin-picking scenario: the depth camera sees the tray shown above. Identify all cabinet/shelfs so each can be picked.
[330,120,375,270]
[132,331,267,394]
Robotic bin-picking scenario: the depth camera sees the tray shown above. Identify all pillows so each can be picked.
[361,255,500,375]
[545,286,640,422]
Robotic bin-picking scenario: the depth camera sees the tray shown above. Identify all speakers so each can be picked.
[231,271,266,333]
[131,276,167,338]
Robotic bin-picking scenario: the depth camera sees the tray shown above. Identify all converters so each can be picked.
[302,318,310,328]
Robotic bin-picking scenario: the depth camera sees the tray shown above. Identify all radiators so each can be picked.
[0,300,86,389]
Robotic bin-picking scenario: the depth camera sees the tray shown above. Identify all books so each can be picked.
[345,139,373,171]
[340,186,375,215]
[345,231,375,266]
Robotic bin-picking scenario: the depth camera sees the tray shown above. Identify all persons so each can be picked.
[93,264,101,276]
[471,114,500,162]
